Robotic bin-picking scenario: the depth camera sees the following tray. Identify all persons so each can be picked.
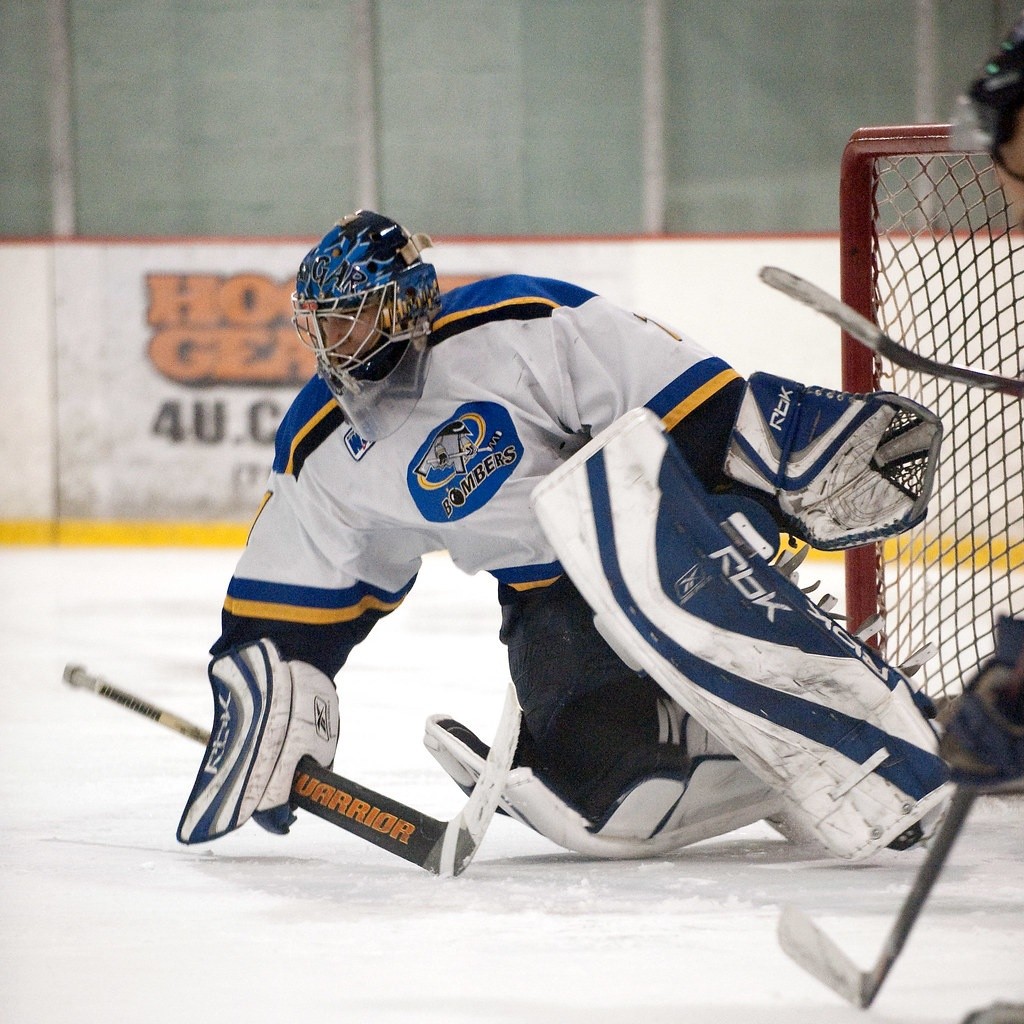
[211,211,946,858]
[929,20,1024,796]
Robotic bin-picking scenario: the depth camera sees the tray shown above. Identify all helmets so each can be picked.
[965,21,1024,146]
[296,209,441,389]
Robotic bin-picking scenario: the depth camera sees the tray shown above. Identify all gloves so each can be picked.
[937,659,1022,795]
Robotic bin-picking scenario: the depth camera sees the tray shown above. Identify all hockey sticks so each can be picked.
[775,659,1024,1008]
[37,638,520,880]
[757,265,1022,396]
